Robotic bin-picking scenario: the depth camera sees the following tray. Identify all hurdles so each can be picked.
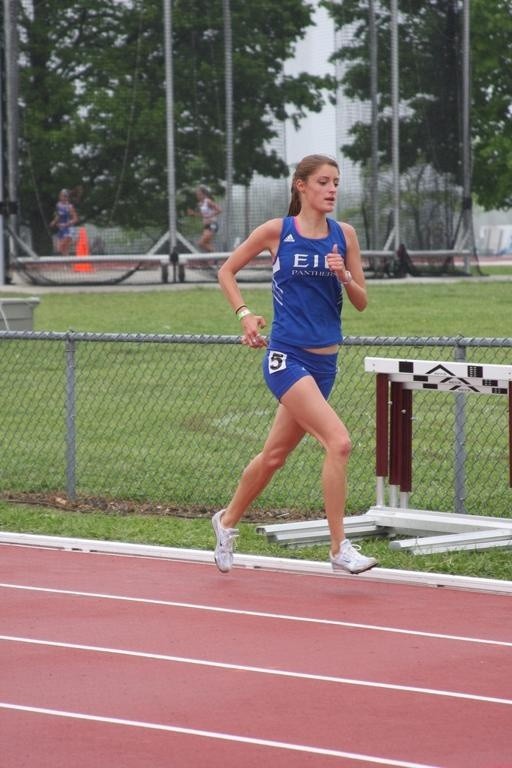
[254,357,512,555]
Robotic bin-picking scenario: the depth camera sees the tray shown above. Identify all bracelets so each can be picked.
[337,270,353,285]
[233,303,248,314]
[233,310,251,320]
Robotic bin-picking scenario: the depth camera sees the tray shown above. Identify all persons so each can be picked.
[48,191,79,257]
[185,189,222,253]
[211,151,393,576]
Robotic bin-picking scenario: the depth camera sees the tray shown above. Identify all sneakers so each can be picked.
[329,539,378,575]
[211,510,239,573]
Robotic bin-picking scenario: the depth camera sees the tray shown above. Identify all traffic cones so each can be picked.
[68,225,97,273]
